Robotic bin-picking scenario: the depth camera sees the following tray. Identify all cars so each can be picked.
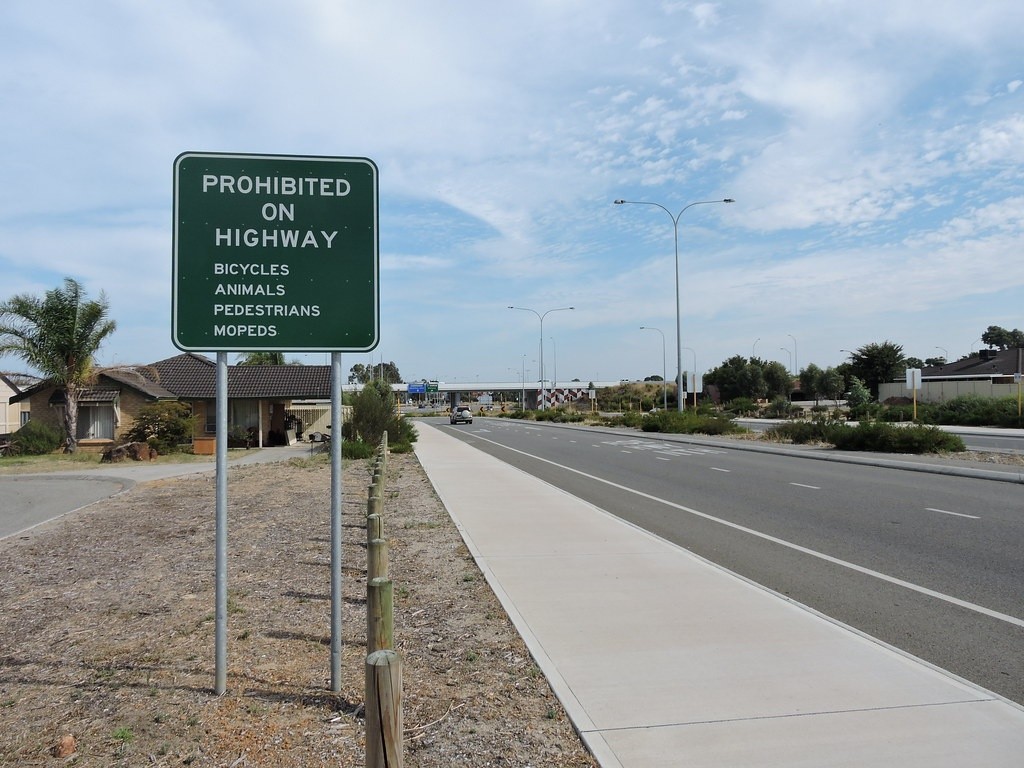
[449,406,473,425]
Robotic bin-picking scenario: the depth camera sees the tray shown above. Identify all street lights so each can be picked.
[936,345,948,359]
[639,326,668,412]
[508,304,577,414]
[751,334,797,375]
[613,198,736,412]
